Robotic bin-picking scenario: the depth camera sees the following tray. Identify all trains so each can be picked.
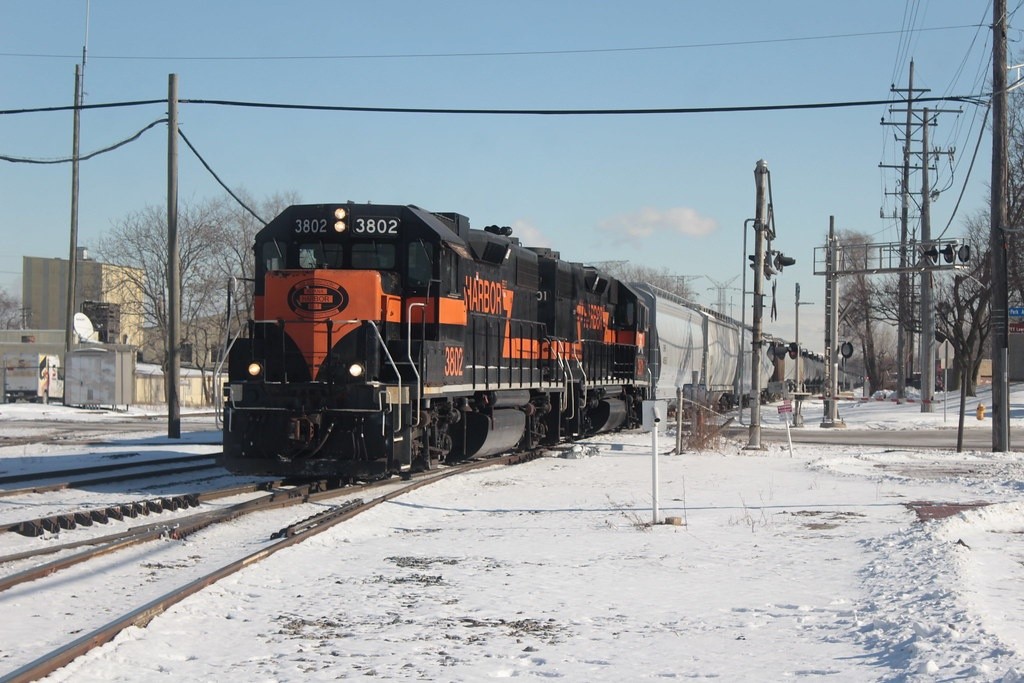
[215,200,865,479]
